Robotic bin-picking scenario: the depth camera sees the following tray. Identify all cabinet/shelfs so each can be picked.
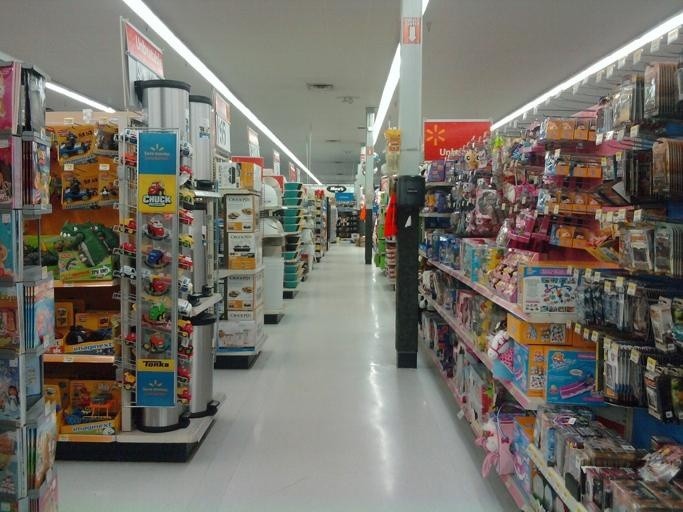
[217,155,269,357]
[336,206,359,239]
[527,61,682,512]
[418,114,606,512]
[0,49,60,512]
[261,175,328,310]
[46,110,230,445]
[327,197,339,242]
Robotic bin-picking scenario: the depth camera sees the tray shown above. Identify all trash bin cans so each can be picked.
[134,78,191,197]
[191,202,212,298]
[178,201,202,306]
[188,95,215,192]
[135,398,191,434]
[180,312,218,417]
